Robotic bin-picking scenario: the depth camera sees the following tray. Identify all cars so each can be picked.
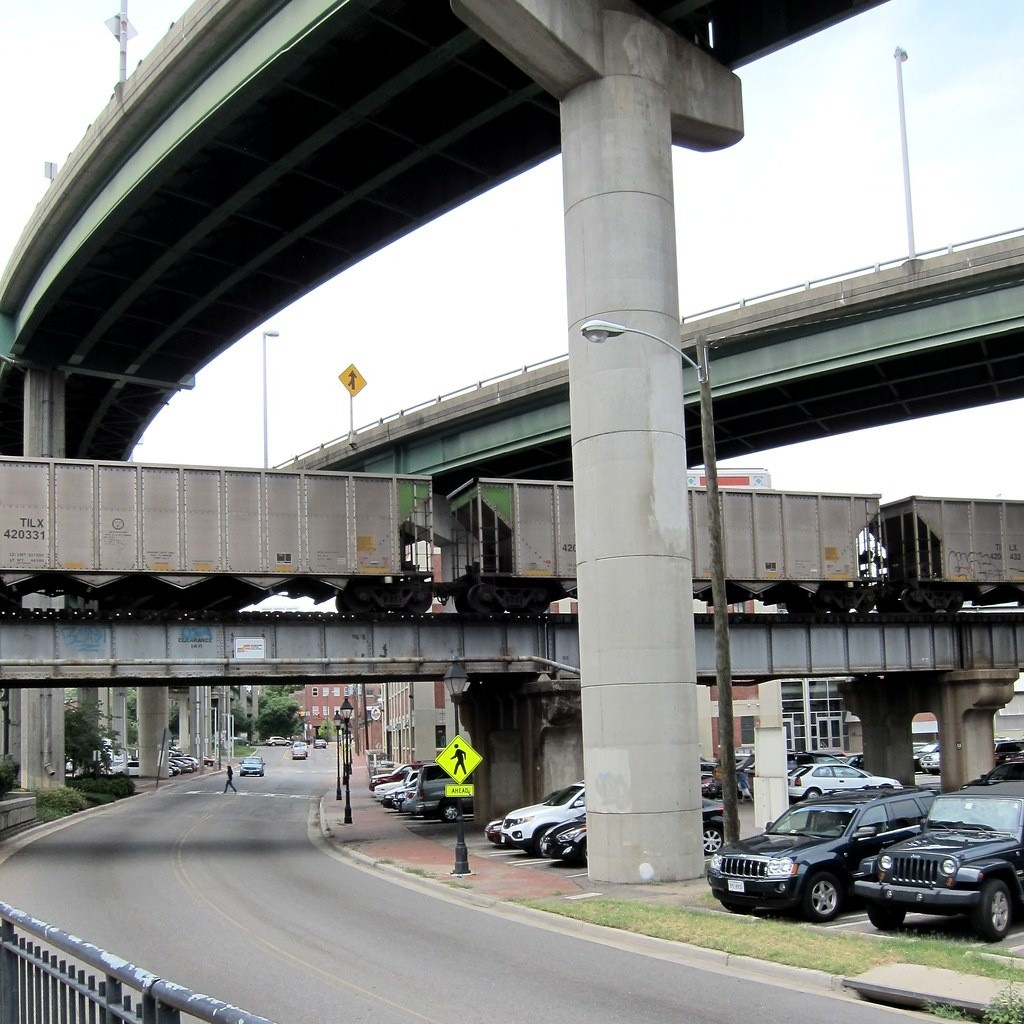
[238,756,266,777]
[115,746,218,778]
[292,738,327,760]
[786,761,902,801]
[369,757,437,823]
[488,739,1024,855]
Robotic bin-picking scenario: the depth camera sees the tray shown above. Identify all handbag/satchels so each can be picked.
[738,783,742,791]
[745,780,751,788]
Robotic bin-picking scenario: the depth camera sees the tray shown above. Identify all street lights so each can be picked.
[332,694,356,823]
[579,315,746,851]
[261,328,280,470]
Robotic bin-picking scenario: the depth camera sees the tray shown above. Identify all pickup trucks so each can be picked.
[265,736,293,747]
[704,787,937,923]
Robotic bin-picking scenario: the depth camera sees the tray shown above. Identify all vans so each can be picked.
[416,763,473,822]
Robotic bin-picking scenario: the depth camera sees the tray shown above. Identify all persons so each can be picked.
[713,759,723,799]
[224,766,237,793]
[739,768,754,803]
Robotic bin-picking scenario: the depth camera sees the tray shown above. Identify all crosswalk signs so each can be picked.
[437,736,483,783]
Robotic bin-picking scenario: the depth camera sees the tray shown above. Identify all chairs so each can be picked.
[816,813,838,828]
[823,768,830,775]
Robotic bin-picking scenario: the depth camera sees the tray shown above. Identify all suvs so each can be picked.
[851,780,1024,943]
